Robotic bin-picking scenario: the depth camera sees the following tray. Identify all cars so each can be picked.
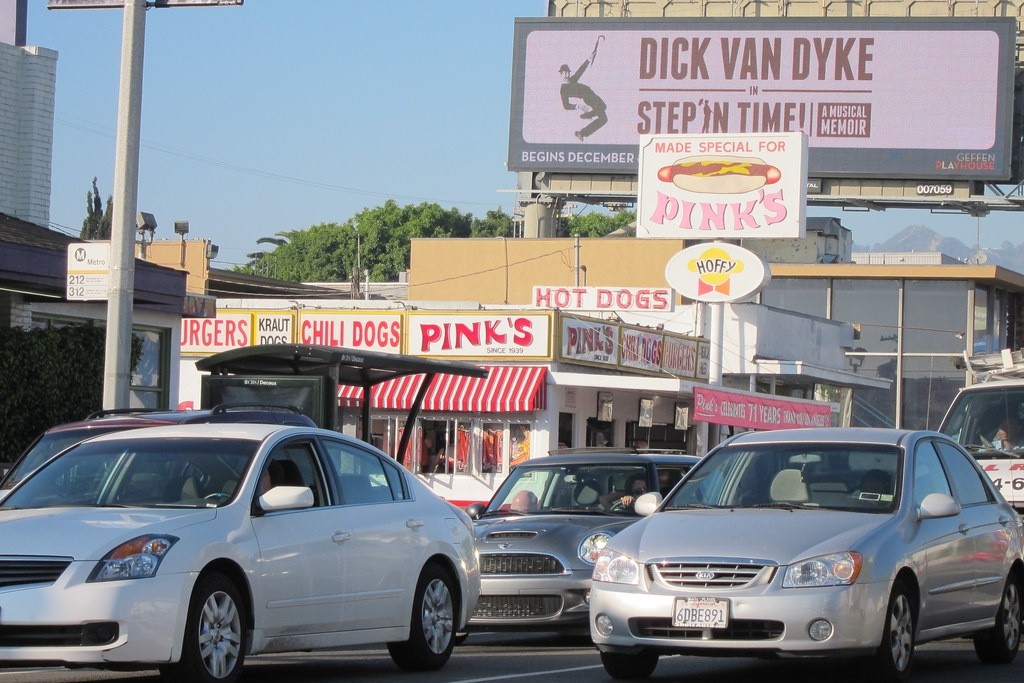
[455,446,726,642]
[589,427,1024,683]
[0,423,482,683]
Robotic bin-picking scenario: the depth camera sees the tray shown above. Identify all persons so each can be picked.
[601,475,647,510]
[977,414,1024,457]
[861,468,889,505]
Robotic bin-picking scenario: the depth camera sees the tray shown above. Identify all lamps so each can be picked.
[861,323,965,339]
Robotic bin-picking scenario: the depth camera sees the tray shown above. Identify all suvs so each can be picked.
[0,401,339,525]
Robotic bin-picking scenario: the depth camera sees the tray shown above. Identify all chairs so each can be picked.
[771,468,812,504]
[573,481,605,509]
[860,469,890,503]
[260,459,305,495]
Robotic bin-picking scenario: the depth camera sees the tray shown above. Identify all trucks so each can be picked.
[934,349,1024,517]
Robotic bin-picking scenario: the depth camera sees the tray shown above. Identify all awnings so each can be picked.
[338,366,549,412]
